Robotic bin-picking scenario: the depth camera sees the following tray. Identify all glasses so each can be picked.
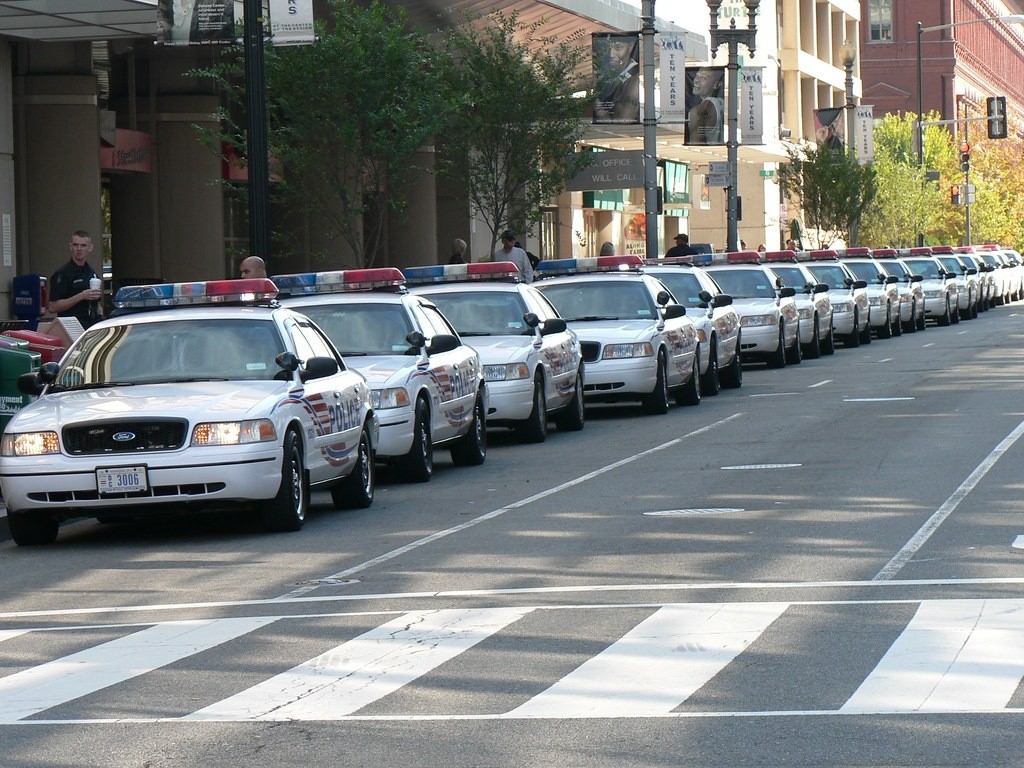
[241,269,251,275]
[506,237,516,241]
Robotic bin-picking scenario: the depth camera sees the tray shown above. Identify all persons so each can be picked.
[758,243,767,252]
[515,240,539,271]
[493,229,534,284]
[786,239,801,252]
[664,233,699,257]
[687,67,724,144]
[599,242,620,271]
[440,237,468,263]
[593,35,641,123]
[47,229,101,332]
[240,255,267,278]
[741,240,747,250]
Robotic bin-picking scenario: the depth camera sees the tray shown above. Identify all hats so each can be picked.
[674,234,689,243]
[501,230,513,238]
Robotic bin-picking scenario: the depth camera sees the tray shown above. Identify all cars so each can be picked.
[616,245,1024,395]
[267,267,489,484]
[530,255,702,415]
[403,260,586,443]
[0,277,379,547]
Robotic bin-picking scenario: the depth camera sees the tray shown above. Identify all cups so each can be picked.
[90,278,102,290]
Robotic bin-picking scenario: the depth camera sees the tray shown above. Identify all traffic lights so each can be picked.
[948,186,960,204]
[959,142,972,176]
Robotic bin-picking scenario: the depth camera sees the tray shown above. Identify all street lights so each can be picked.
[838,38,856,247]
[914,14,1023,244]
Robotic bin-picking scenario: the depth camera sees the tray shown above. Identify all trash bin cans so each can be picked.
[119,278,166,286]
